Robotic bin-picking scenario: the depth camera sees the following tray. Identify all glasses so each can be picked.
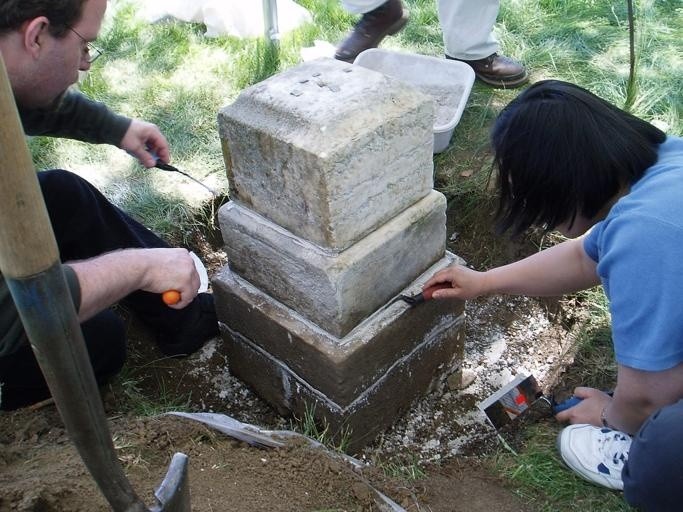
[65,25,104,64]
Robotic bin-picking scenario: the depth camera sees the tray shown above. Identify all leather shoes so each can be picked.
[445,54,528,89]
[336,1,409,60]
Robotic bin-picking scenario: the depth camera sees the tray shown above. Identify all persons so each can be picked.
[333,0,528,91]
[0,0,218,413]
[422,81,683,512]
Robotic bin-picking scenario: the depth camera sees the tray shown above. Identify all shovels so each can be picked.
[0,54,192,512]
[383,282,452,320]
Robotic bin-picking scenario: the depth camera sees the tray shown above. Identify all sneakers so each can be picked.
[557,423,632,489]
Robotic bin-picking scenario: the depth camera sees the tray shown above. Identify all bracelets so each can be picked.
[600,403,609,428]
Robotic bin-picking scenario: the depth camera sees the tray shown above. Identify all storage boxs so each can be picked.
[352,47,477,155]
